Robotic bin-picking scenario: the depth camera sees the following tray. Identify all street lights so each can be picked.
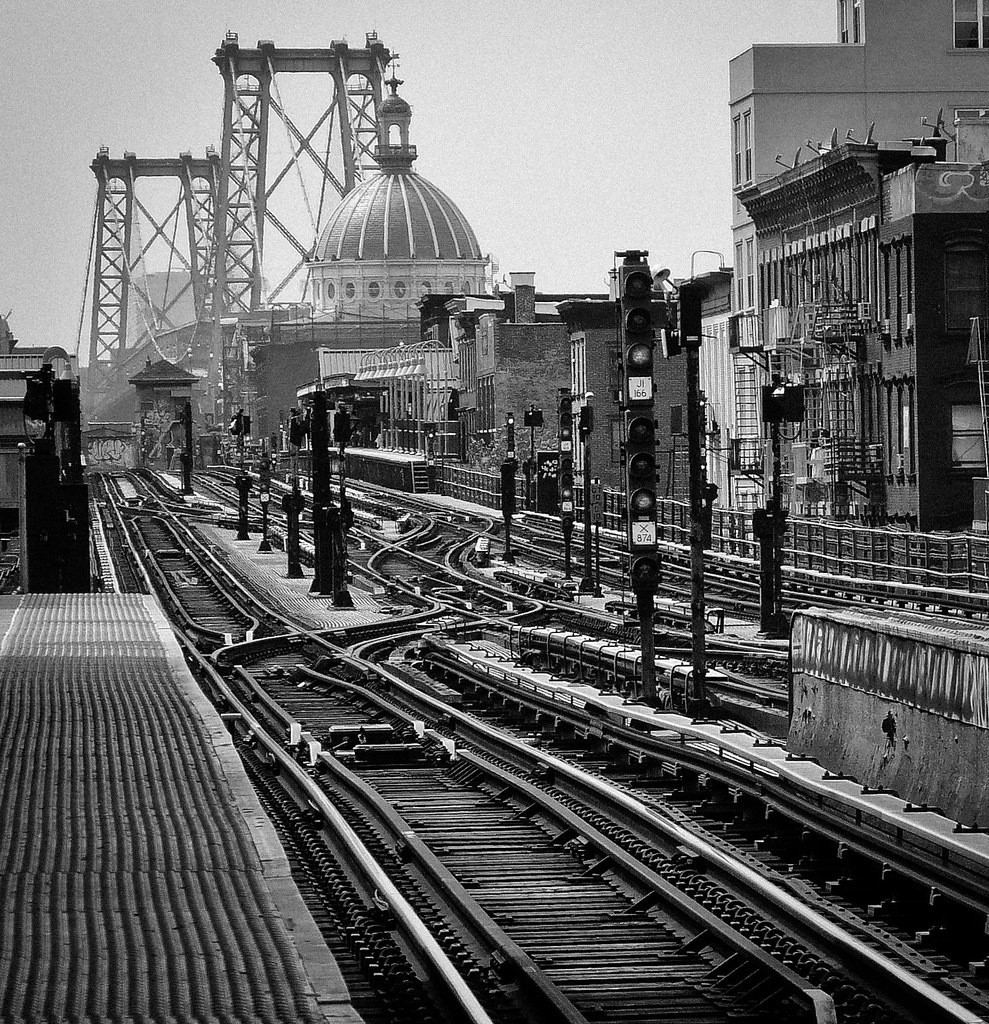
[354,340,454,461]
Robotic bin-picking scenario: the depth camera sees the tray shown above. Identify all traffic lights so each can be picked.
[750,371,822,642]
[501,266,723,595]
[221,383,356,612]
